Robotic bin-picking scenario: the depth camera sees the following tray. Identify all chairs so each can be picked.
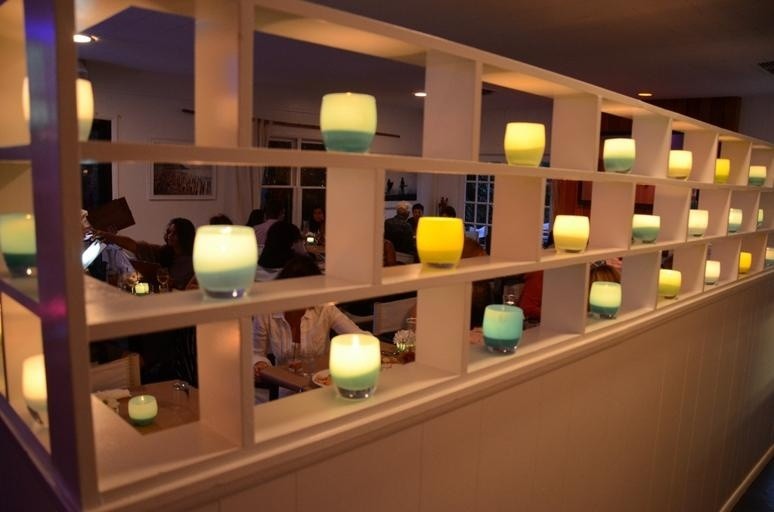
[396,251,415,263]
[339,297,416,336]
[90,352,141,392]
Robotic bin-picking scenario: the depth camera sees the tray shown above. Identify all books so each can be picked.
[87,196,136,233]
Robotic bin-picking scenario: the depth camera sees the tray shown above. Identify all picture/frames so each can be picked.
[144,135,217,200]
[576,133,721,210]
[384,170,424,209]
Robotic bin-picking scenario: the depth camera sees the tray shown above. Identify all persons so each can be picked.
[250,256,375,386]
[93,217,196,290]
[256,223,308,282]
[382,199,413,239]
[407,203,424,228]
[251,202,285,249]
[302,203,326,237]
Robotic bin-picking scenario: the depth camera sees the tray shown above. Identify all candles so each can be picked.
[588,281,621,322]
[1,213,38,280]
[483,304,524,355]
[191,224,258,300]
[328,334,381,401]
[319,91,379,153]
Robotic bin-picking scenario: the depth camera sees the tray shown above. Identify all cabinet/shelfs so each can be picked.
[1,0,774,512]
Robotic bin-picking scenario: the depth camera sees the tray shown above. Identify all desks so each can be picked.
[258,341,416,394]
[92,379,200,436]
